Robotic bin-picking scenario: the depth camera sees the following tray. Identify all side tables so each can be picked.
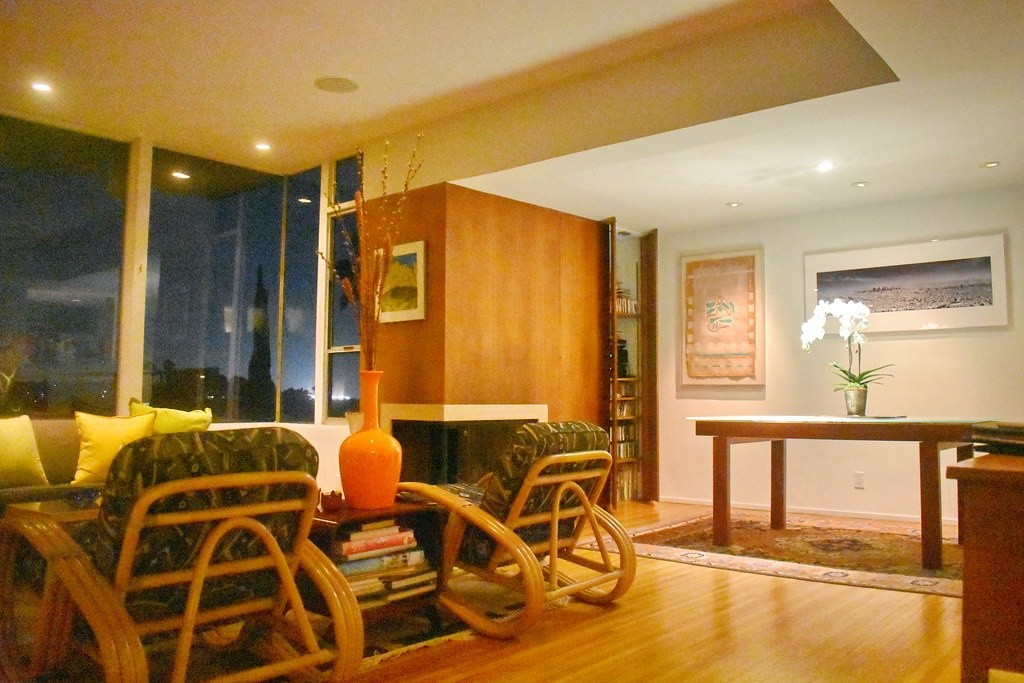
[309,499,451,640]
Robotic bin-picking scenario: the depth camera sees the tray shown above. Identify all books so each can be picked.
[324,516,437,611]
[971,421,1024,456]
[616,298,638,313]
[610,382,639,501]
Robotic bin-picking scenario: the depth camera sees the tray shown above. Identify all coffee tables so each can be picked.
[8,495,100,527]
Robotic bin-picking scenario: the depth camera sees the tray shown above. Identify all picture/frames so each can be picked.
[804,234,1009,334]
[374,241,425,323]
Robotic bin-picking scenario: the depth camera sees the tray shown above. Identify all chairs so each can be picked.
[0,427,366,682]
[395,420,635,641]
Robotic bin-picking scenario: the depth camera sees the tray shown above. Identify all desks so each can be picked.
[696,419,996,570]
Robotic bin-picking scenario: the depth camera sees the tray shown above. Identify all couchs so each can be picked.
[0,419,106,515]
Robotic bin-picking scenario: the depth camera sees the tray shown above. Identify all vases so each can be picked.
[338,370,403,509]
[844,387,868,416]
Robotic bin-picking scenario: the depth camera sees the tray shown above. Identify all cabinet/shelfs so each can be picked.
[946,454,1024,683]
[362,183,659,510]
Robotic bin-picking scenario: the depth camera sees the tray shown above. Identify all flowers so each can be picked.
[310,133,425,372]
[801,298,895,392]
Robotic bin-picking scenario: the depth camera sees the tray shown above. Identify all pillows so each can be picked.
[72,411,157,485]
[128,397,213,436]
[0,415,48,488]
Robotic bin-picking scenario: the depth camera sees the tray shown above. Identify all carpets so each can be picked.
[577,512,965,599]
[31,561,570,683]
